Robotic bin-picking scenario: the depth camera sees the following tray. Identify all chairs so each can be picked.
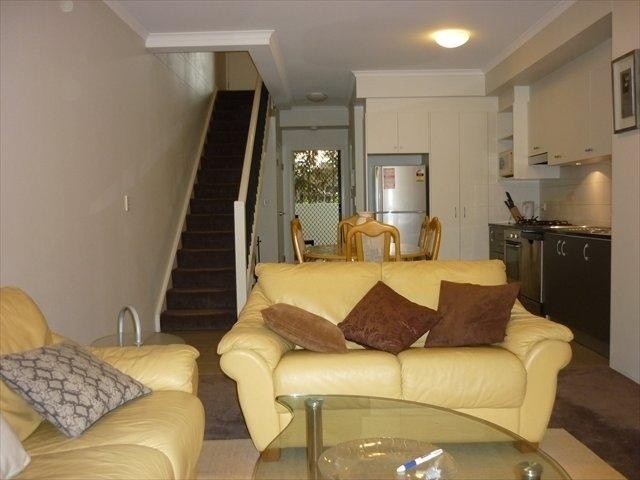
[290,213,442,263]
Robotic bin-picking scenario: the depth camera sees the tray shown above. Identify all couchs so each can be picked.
[0,285,206,480]
[216,258,576,462]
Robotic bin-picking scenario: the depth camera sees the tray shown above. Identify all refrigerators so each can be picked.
[375,165,427,255]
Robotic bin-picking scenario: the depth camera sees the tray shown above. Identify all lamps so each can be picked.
[431,29,471,49]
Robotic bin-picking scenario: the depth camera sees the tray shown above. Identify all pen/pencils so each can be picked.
[396,448,444,474]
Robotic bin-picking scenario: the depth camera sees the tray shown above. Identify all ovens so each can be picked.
[503,227,543,304]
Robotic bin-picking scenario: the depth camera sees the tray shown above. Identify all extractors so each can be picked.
[527,152,548,167]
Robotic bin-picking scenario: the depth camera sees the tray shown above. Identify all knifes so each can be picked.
[502,192,514,209]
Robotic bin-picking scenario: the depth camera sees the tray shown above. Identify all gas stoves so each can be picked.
[517,216,577,232]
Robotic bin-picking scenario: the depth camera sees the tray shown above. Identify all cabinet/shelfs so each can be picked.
[430,112,488,259]
[495,85,562,180]
[526,81,547,157]
[576,236,611,344]
[547,235,575,330]
[365,112,430,153]
[546,61,611,166]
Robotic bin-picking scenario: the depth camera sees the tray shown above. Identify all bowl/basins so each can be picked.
[317,436,461,480]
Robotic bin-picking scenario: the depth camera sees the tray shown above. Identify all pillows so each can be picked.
[0,340,153,439]
[424,279,524,348]
[260,303,347,353]
[0,415,31,479]
[336,280,443,354]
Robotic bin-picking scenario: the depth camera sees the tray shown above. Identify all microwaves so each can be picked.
[498,150,513,177]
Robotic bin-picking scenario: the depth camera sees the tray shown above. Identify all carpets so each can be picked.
[187,427,630,480]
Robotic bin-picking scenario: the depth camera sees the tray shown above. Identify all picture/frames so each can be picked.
[612,49,640,134]
